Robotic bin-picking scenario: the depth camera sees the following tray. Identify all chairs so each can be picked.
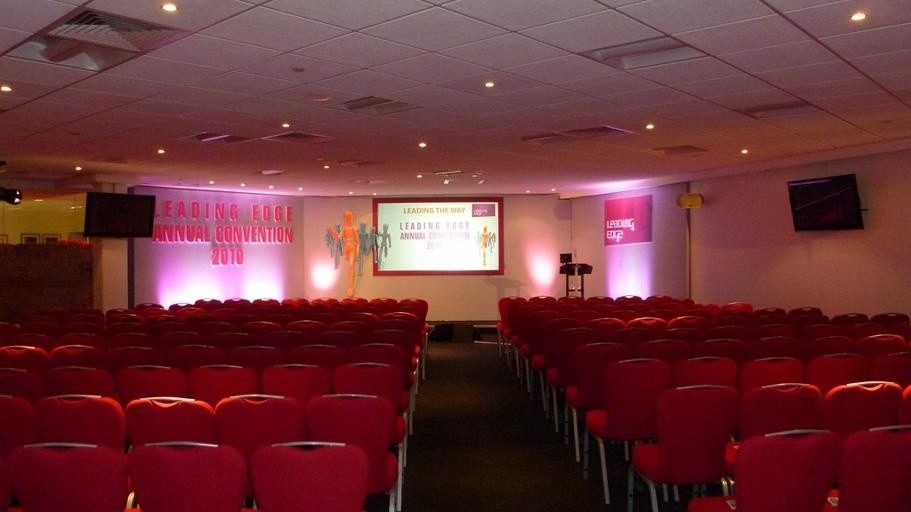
[213,333,259,348]
[106,308,131,316]
[83,308,106,319]
[900,384,910,424]
[342,312,378,327]
[616,295,642,303]
[808,352,872,394]
[299,303,328,310]
[849,321,886,337]
[112,333,159,346]
[342,297,369,306]
[826,380,901,436]
[281,298,310,307]
[647,295,673,303]
[582,357,671,504]
[108,346,163,370]
[237,303,262,310]
[740,356,806,390]
[799,323,846,339]
[363,301,394,313]
[789,314,829,327]
[331,302,361,311]
[520,310,565,393]
[723,302,754,311]
[673,356,738,502]
[349,343,414,468]
[731,383,825,474]
[673,299,695,308]
[380,311,425,411]
[827,425,910,511]
[262,332,311,349]
[332,321,374,331]
[1,395,33,451]
[333,362,409,511]
[805,335,860,356]
[319,331,368,347]
[223,298,251,306]
[394,303,426,394]
[371,298,398,306]
[305,394,398,512]
[253,298,281,308]
[212,304,235,309]
[645,309,682,321]
[126,442,249,512]
[752,324,796,337]
[262,364,332,403]
[109,322,145,333]
[668,316,712,329]
[198,322,236,332]
[870,312,911,325]
[502,301,532,368]
[228,346,287,370]
[530,317,583,412]
[288,344,348,369]
[148,315,181,325]
[869,351,911,387]
[754,307,788,318]
[830,312,869,325]
[168,345,223,371]
[216,394,306,459]
[286,320,329,330]
[63,321,102,334]
[50,345,107,370]
[564,342,635,462]
[20,322,61,338]
[684,309,714,321]
[529,296,557,302]
[1,367,45,401]
[33,395,126,449]
[195,298,222,308]
[634,338,692,354]
[587,304,616,311]
[59,333,106,346]
[371,329,421,436]
[607,310,640,322]
[135,303,163,310]
[13,333,56,350]
[189,364,261,406]
[311,298,340,306]
[627,318,667,334]
[176,307,209,320]
[125,397,217,446]
[0,345,49,372]
[301,312,340,326]
[160,332,210,344]
[558,296,586,303]
[621,304,651,311]
[399,298,435,380]
[891,321,910,337]
[70,313,104,324]
[140,308,174,318]
[511,303,546,379]
[553,304,583,313]
[152,322,190,331]
[496,296,528,357]
[108,313,144,324]
[268,303,294,310]
[718,309,752,321]
[224,315,257,324]
[586,296,614,304]
[607,328,657,347]
[240,321,283,334]
[658,328,712,345]
[628,383,742,512]
[691,303,721,311]
[261,314,299,323]
[169,303,194,313]
[659,303,687,312]
[116,364,190,403]
[45,366,119,398]
[186,315,221,324]
[859,333,906,353]
[566,310,603,323]
[787,307,822,318]
[711,326,750,338]
[586,317,625,335]
[250,442,370,510]
[692,338,748,358]
[545,327,595,432]
[6,443,136,512]
[688,429,840,509]
[750,336,806,358]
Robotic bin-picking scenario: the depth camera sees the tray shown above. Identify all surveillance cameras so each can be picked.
[1,187,24,205]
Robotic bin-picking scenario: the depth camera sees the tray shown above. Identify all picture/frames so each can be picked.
[604,194,652,245]
[372,197,504,276]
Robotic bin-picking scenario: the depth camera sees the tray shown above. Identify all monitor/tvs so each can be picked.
[83,191,156,237]
[786,174,864,233]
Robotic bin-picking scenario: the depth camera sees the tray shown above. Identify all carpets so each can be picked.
[365,325,724,512]
